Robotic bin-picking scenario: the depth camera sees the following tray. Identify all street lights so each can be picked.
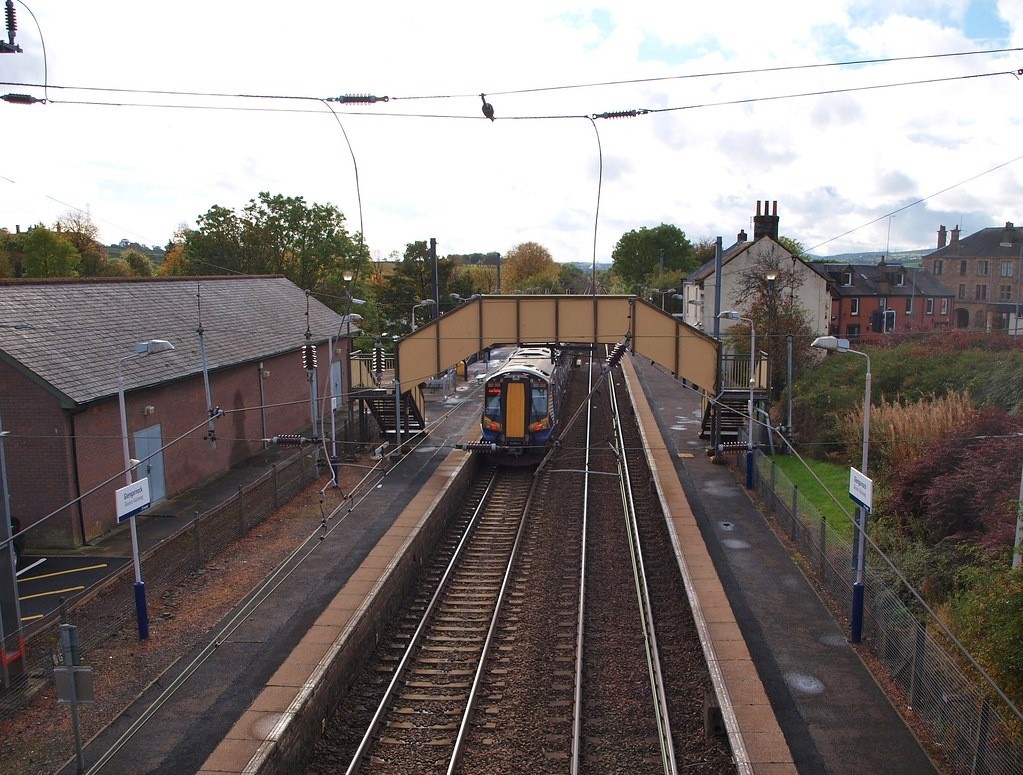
[411,299,436,332]
[762,271,778,429]
[717,311,755,491]
[449,292,476,304]
[651,287,677,311]
[810,336,872,645]
[116,339,178,639]
[341,267,355,454]
[908,266,925,334]
[327,313,364,488]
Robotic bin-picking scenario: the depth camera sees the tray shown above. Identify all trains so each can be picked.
[478,341,577,468]
